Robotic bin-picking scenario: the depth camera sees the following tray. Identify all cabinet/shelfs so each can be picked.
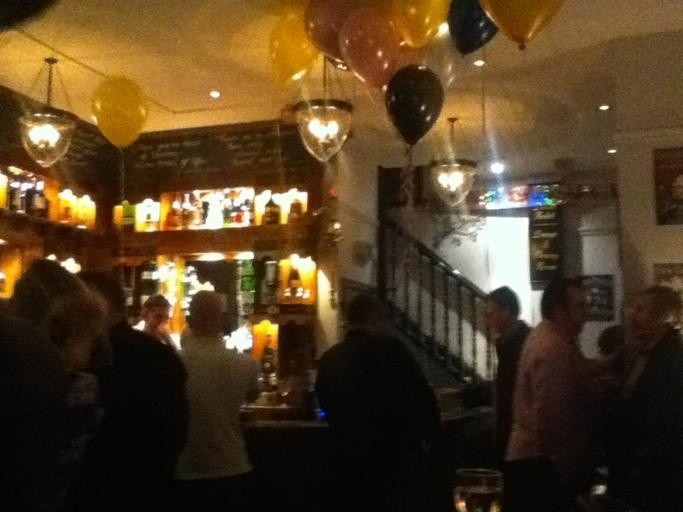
[114,186,319,416]
[0,165,126,310]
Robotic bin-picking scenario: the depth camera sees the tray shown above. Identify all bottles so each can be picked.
[288,266,300,296]
[263,260,279,303]
[0,170,96,233]
[107,185,302,233]
[259,336,279,385]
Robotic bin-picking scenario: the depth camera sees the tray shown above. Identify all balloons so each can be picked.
[92,78,148,148]
[269,1,564,147]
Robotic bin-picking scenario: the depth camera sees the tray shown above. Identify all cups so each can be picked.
[454,468,503,512]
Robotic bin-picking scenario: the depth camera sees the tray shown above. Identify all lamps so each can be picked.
[432,119,476,209]
[289,56,355,165]
[20,59,76,168]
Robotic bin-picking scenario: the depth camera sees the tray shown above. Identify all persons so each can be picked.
[452,277,682,511]
[315,291,441,512]
[0,258,260,511]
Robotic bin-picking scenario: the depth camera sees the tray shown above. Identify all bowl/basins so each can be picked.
[247,403,293,421]
